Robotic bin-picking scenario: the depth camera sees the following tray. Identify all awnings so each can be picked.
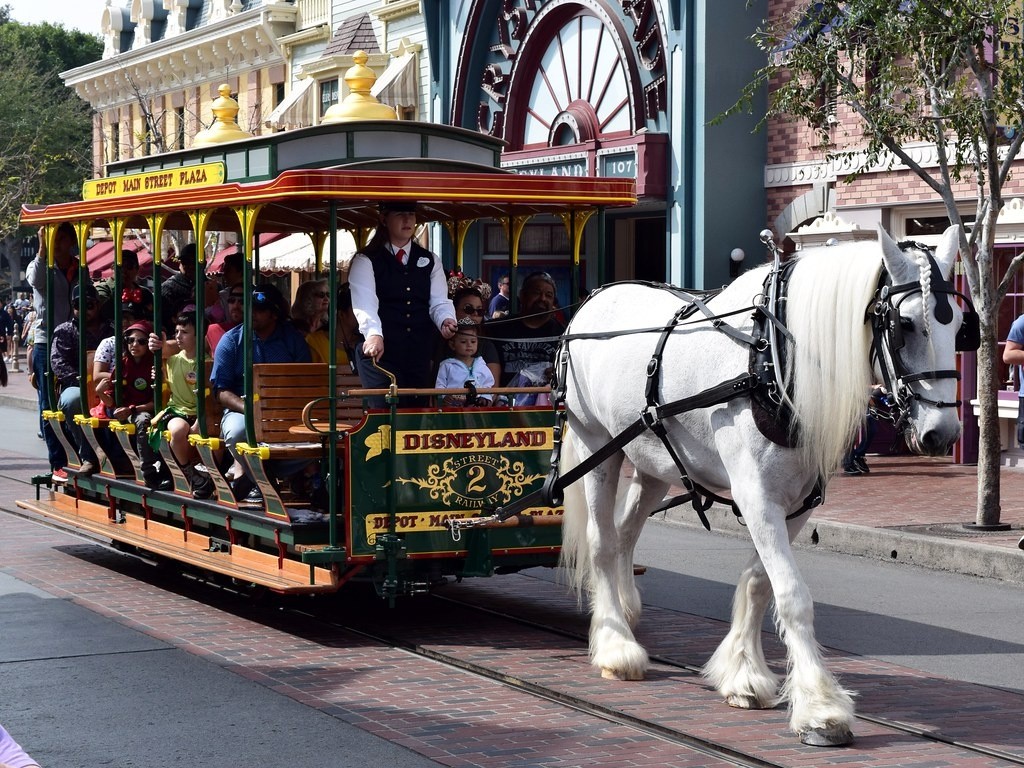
[768,0,919,67]
[204,223,427,276]
[74,241,113,265]
[87,238,149,278]
[264,78,314,130]
[101,244,174,278]
[370,53,415,107]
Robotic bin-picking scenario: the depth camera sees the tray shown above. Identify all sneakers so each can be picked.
[52,468,68,482]
[182,462,208,488]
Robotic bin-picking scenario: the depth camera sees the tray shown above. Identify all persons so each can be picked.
[347,200,459,423]
[843,397,876,475]
[50,244,364,513]
[488,274,521,320]
[484,271,566,407]
[26,222,90,483]
[424,288,500,407]
[1003,314,1024,550]
[0,292,37,387]
[434,317,495,406]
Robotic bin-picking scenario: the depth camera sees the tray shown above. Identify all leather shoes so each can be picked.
[231,484,264,502]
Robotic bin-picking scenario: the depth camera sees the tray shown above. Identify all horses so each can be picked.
[555,223,963,749]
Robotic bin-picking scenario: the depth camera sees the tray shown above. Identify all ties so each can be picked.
[396,249,406,263]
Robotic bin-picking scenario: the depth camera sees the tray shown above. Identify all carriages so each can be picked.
[16,48,983,749]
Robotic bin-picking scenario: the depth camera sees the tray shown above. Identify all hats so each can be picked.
[521,271,556,298]
[122,302,145,320]
[250,284,282,311]
[72,284,99,301]
[178,243,207,262]
[378,200,417,212]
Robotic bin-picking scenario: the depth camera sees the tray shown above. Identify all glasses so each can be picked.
[226,296,242,304]
[313,291,330,299]
[502,282,509,286]
[458,306,485,316]
[123,336,147,346]
[123,264,140,271]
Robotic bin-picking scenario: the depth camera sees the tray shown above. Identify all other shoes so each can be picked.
[145,475,171,489]
[844,465,863,474]
[79,459,99,474]
[852,455,870,473]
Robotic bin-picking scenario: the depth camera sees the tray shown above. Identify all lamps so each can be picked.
[730,248,745,280]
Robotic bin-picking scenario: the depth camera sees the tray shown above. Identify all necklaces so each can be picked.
[336,336,344,343]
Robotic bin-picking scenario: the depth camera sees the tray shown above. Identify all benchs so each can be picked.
[85,340,366,457]
